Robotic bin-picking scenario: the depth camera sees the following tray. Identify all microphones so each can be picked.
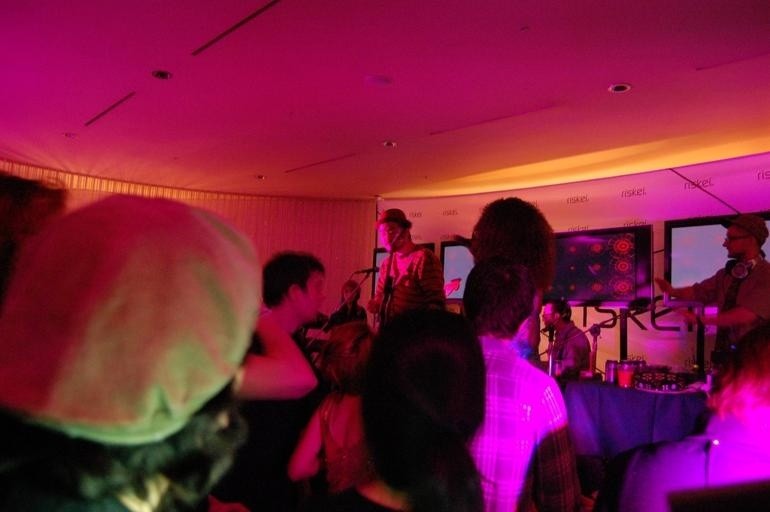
[353,267,380,274]
[541,307,571,333]
[389,220,412,248]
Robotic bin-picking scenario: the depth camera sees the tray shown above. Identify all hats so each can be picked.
[719,211,769,244]
[0,193,265,451]
[373,207,414,232]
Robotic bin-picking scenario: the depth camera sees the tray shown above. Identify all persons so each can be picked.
[1,163,770,512]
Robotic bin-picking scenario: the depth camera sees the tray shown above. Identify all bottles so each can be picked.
[604,358,645,389]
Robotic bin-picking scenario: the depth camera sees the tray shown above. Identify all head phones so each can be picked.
[724,249,766,279]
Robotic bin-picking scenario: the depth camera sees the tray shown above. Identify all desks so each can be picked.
[567,380,705,457]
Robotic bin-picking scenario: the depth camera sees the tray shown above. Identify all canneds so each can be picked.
[605,360,645,387]
